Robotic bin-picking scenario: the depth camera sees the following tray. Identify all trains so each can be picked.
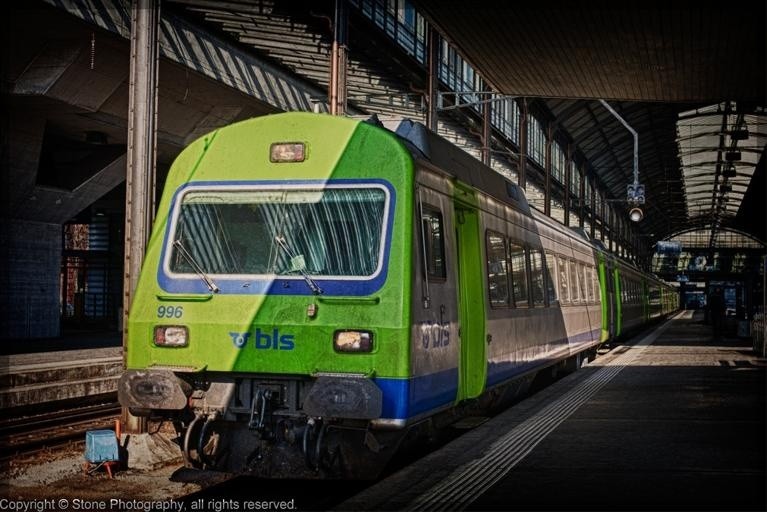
[115,104,680,496]
